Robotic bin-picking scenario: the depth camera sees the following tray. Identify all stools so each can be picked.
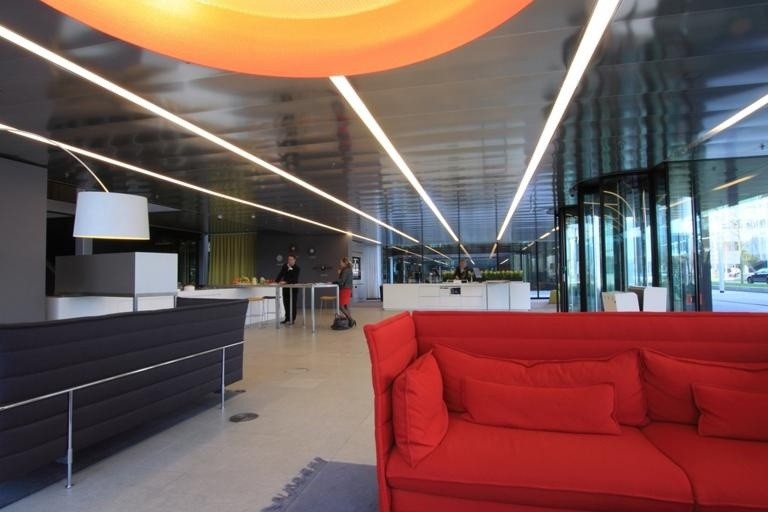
[316,295,336,313]
[262,295,281,321]
[246,296,264,327]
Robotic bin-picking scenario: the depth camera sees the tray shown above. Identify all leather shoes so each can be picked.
[280,318,295,325]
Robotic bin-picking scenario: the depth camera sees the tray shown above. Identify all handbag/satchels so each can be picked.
[330,317,349,330]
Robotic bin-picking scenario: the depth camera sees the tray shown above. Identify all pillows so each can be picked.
[458,376,622,437]
[388,350,448,466]
[690,382,766,445]
[432,341,647,430]
[637,346,767,425]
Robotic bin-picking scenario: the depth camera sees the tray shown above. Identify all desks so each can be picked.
[237,278,338,333]
[627,283,668,313]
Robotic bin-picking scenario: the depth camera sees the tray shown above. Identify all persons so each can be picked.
[275,254,300,325]
[453,259,468,283]
[327,257,357,328]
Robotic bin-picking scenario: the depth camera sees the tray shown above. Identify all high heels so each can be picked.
[349,320,358,328]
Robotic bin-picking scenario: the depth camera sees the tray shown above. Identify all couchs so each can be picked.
[364,305,766,512]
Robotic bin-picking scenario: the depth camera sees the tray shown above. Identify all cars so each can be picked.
[746,268,768,285]
[725,268,741,278]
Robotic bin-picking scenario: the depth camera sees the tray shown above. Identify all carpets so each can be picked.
[263,455,379,512]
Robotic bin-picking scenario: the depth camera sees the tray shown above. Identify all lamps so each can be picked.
[45,139,153,242]
